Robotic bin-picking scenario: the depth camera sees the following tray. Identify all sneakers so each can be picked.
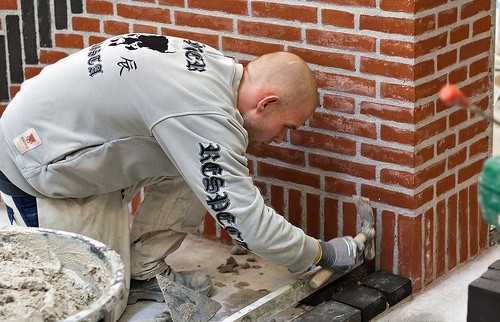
[127,267,214,305]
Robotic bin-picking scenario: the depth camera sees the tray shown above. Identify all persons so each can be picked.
[1,33,366,304]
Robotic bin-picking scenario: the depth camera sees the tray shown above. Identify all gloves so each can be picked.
[315,235,365,271]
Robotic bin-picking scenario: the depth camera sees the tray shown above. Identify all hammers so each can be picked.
[311,195,375,289]
